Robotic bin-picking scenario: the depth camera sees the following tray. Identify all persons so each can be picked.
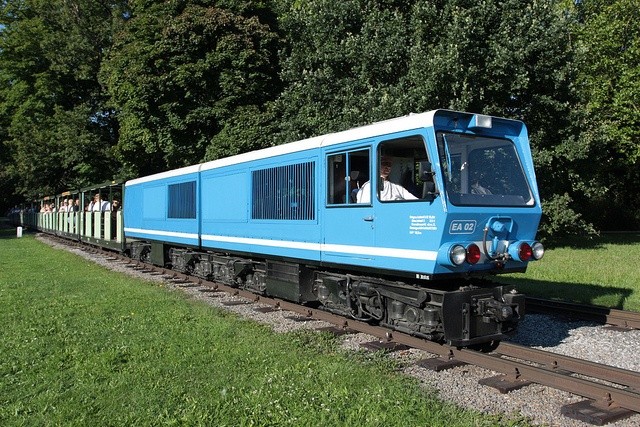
[38,193,119,213]
[357,156,419,202]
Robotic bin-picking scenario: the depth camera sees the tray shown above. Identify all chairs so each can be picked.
[349,166,369,204]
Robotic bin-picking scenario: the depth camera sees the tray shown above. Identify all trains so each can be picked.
[9,109,544,349]
